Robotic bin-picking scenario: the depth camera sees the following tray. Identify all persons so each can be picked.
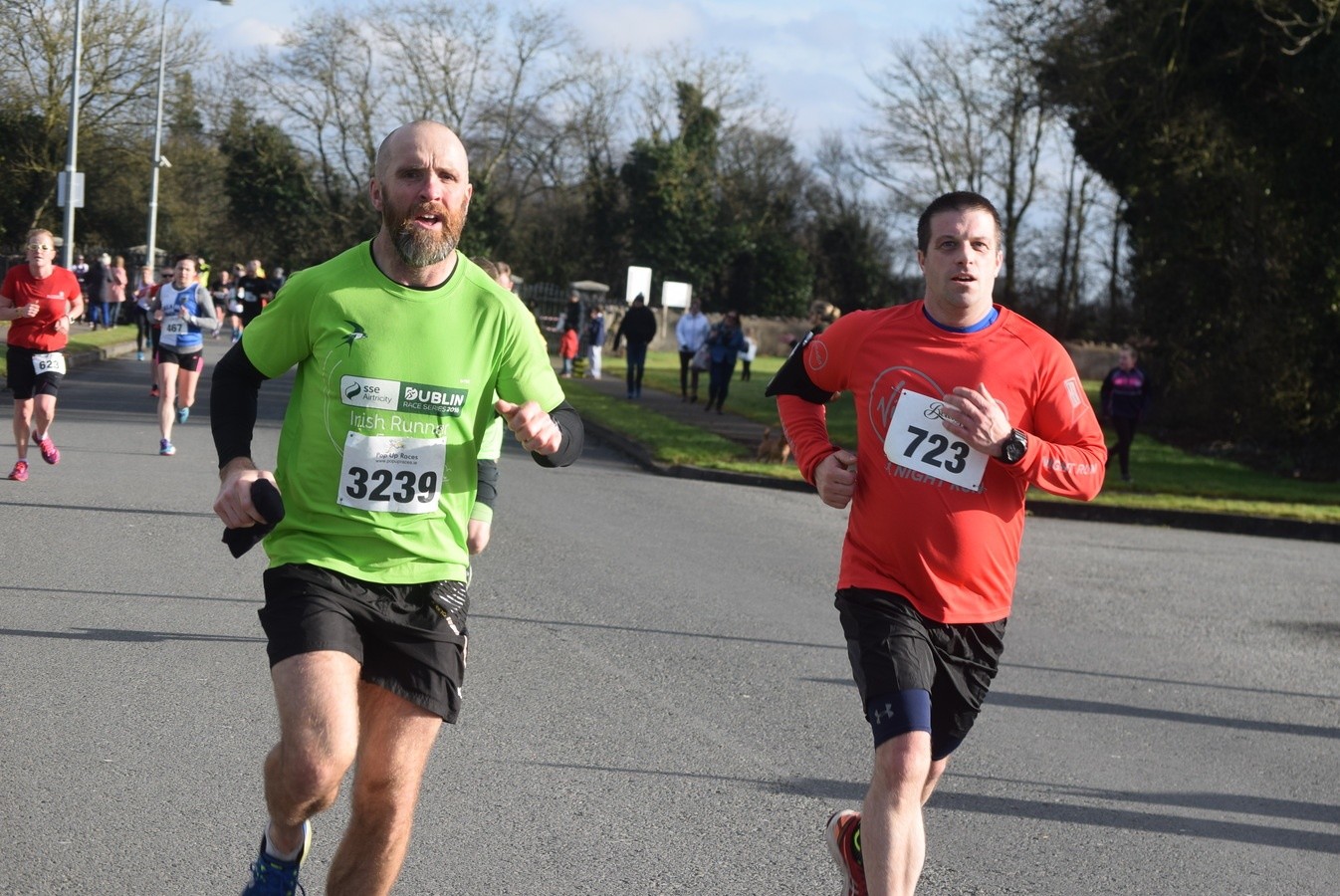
[1097,343,1145,479]
[765,191,1109,896]
[1,229,83,483]
[468,258,841,556]
[146,254,218,456]
[71,252,285,399]
[210,117,582,895]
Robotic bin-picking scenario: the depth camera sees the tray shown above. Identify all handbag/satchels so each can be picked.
[693,350,711,369]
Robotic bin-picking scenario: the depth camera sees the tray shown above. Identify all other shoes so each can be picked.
[136,351,144,363]
[703,399,713,411]
[716,404,724,415]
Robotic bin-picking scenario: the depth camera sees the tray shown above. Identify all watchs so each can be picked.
[994,428,1027,464]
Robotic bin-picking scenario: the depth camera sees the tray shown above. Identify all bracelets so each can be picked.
[65,314,75,324]
[16,306,22,318]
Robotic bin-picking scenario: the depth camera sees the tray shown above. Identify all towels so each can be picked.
[220,478,286,561]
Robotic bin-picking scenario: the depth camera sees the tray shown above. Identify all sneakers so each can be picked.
[159,438,176,456]
[236,811,313,896]
[825,809,869,896]
[8,461,29,482]
[31,431,60,465]
[175,396,189,424]
